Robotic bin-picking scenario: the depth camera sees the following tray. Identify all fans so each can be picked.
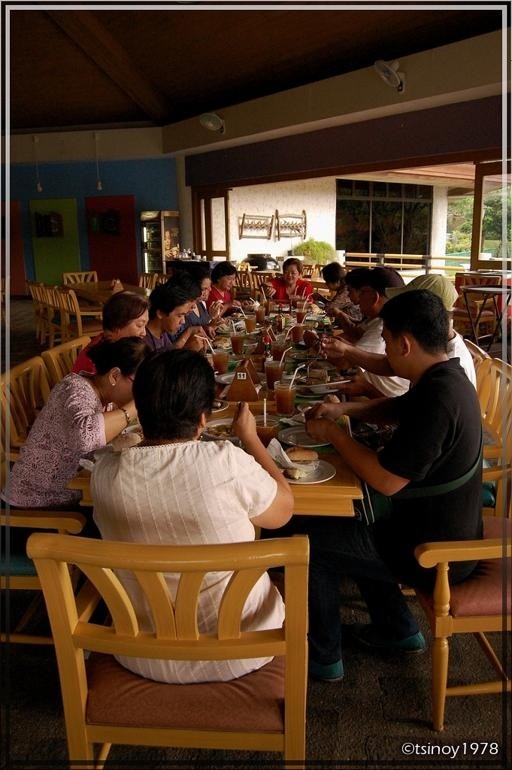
[198,112,227,135]
[372,59,406,94]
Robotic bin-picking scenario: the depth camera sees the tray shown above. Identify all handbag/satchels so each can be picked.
[354,481,393,525]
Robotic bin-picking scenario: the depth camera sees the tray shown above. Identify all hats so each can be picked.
[371,267,403,293]
[385,273,459,310]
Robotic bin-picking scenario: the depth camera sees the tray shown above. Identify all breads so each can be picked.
[287,450,319,479]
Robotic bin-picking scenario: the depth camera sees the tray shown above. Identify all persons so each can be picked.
[0,256,484,685]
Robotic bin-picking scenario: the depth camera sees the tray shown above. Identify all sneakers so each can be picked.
[351,624,428,656]
[309,658,343,682]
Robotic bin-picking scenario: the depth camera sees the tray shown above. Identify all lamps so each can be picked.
[32,138,43,192]
[92,131,104,192]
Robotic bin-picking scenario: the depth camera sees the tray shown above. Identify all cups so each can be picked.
[206,294,310,447]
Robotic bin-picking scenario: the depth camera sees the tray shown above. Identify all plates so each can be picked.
[121,424,142,435]
[279,459,336,484]
[277,425,331,448]
[304,376,352,397]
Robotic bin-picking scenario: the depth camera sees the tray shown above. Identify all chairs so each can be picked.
[399,467,512,735]
[27,533,313,770]
[0,460,97,653]
